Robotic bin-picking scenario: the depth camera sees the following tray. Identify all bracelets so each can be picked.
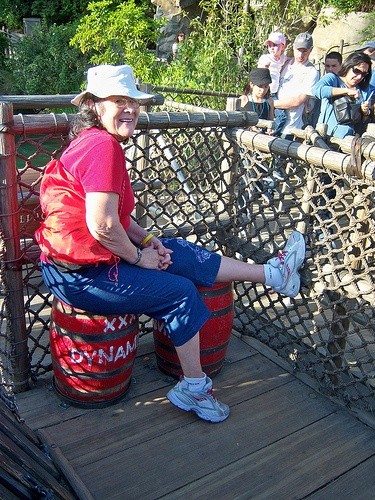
[141,233,153,246]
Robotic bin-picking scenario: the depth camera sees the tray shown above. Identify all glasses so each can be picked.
[351,66,369,77]
[100,97,139,107]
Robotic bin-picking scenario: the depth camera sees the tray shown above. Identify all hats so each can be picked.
[355,40,375,51]
[264,32,285,47]
[294,33,313,50]
[247,68,270,86]
[71,64,155,104]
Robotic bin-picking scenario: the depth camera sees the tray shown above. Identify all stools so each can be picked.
[50,293,139,410]
[151,283,233,382]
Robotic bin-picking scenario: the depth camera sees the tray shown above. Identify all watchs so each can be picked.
[132,248,143,265]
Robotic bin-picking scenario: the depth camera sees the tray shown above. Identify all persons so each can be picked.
[34,65,307,424]
[236,67,275,225]
[268,33,320,187]
[254,31,294,139]
[172,31,186,59]
[357,41,375,83]
[298,51,375,249]
[303,50,346,131]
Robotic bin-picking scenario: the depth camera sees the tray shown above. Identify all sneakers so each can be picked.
[267,230,305,297]
[167,376,231,423]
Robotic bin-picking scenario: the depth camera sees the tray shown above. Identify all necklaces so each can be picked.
[250,94,266,118]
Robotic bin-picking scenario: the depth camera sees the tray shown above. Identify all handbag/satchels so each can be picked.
[334,94,363,124]
